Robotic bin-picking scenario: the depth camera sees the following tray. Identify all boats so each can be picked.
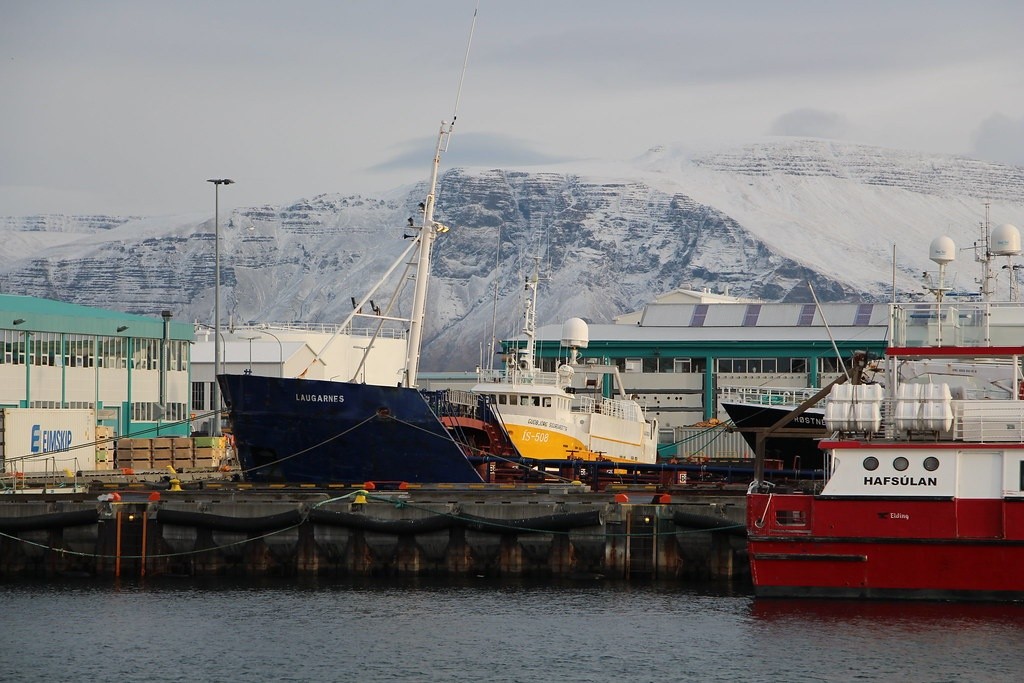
[497,282,1024,460]
[145,481,206,490]
[721,197,1024,597]
[215,10,657,487]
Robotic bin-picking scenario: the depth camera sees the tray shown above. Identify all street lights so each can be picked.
[353,345,375,385]
[207,178,235,437]
[238,337,262,376]
